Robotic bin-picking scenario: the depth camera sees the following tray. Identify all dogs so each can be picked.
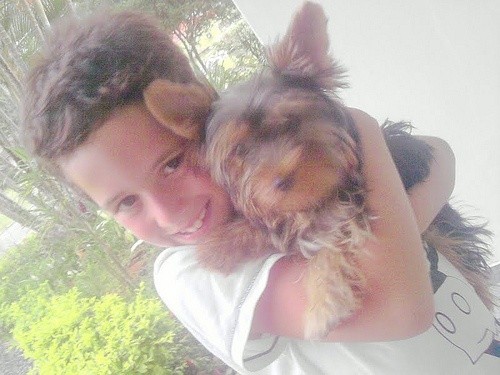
[142,0,500,342]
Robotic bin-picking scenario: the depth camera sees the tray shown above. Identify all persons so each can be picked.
[21,10,500,375]
